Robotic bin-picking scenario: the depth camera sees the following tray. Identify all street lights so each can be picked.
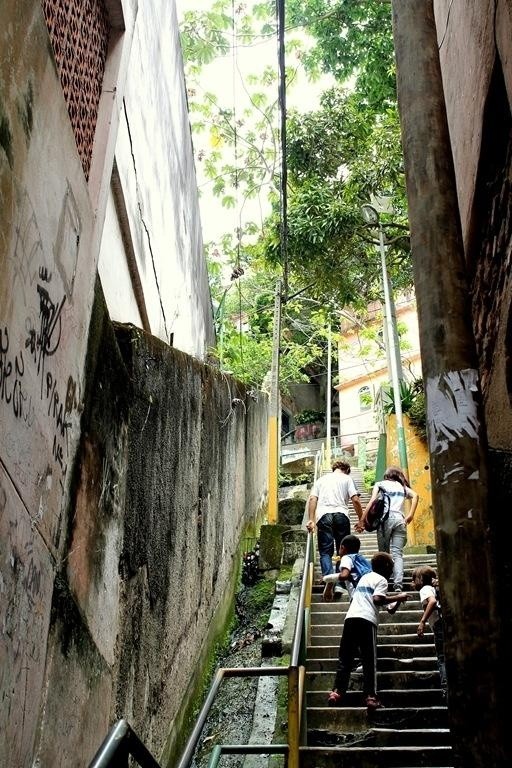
[361,204,416,547]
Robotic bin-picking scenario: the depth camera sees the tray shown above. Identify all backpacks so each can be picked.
[364,482,391,532]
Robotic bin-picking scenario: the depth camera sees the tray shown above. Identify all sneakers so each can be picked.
[323,579,334,602]
[327,688,341,707]
[367,697,385,708]
[395,586,402,592]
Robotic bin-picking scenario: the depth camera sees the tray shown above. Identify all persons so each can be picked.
[306,460,365,602]
[323,535,412,708]
[353,466,419,592]
[413,566,449,702]
